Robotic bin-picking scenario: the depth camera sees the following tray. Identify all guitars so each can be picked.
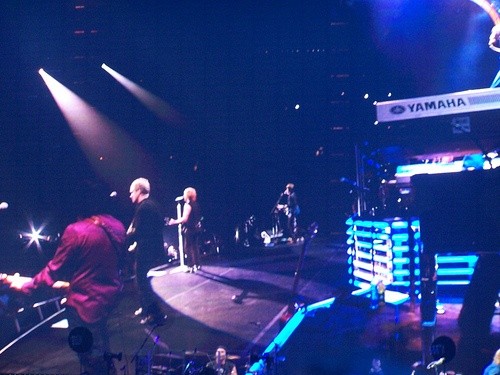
[1,273,70,293]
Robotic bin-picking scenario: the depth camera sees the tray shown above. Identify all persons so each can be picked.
[0,174,133,375]
[148,181,313,261]
[168,185,203,275]
[121,176,167,325]
[205,344,238,374]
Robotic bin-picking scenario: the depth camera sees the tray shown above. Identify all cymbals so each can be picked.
[185,351,208,356]
[138,354,184,374]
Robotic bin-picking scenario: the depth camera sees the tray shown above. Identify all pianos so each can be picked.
[376,87,500,144]
